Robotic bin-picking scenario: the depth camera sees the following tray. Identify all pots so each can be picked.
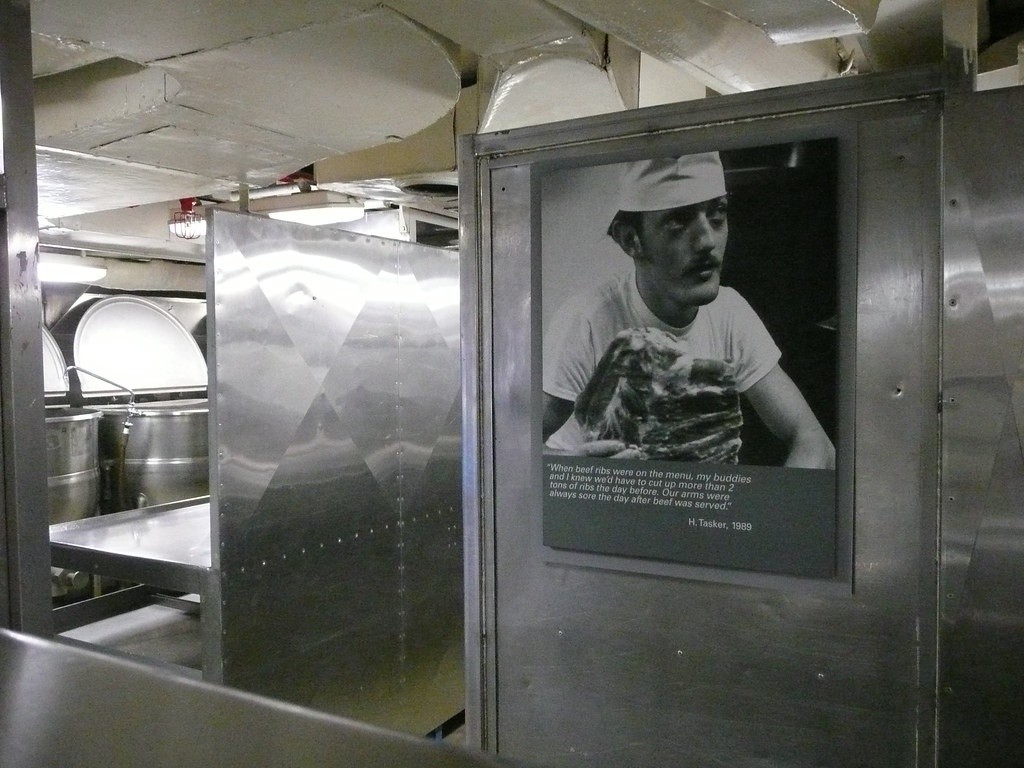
[74,405,209,508]
[41,405,104,525]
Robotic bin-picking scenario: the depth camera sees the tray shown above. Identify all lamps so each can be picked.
[167,191,365,239]
